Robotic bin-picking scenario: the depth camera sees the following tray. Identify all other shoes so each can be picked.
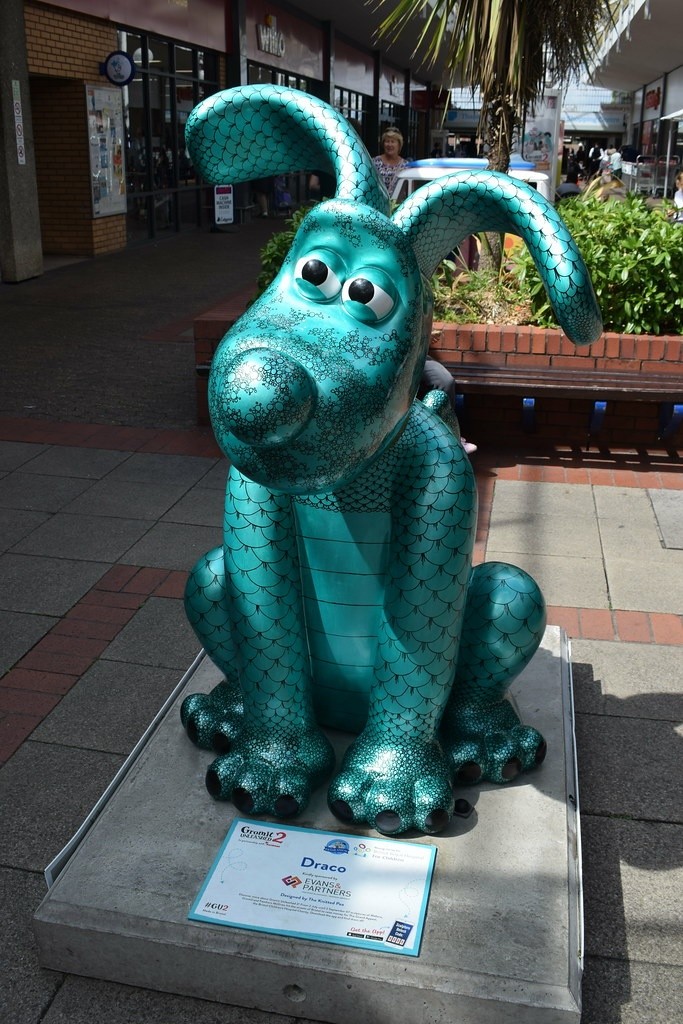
[256,212,269,219]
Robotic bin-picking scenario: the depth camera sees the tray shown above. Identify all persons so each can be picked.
[564,141,623,184]
[371,128,410,204]
[255,180,275,218]
[422,329,478,454]
[666,172,683,221]
